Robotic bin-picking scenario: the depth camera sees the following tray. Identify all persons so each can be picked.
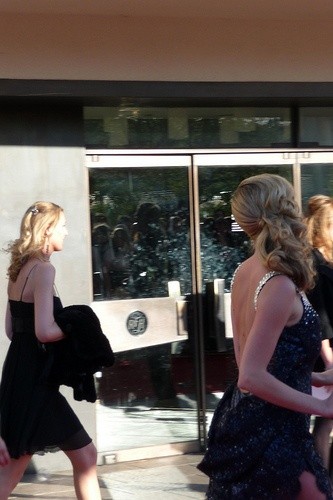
[1,202,104,500]
[198,174,333,500]
[303,194,333,466]
